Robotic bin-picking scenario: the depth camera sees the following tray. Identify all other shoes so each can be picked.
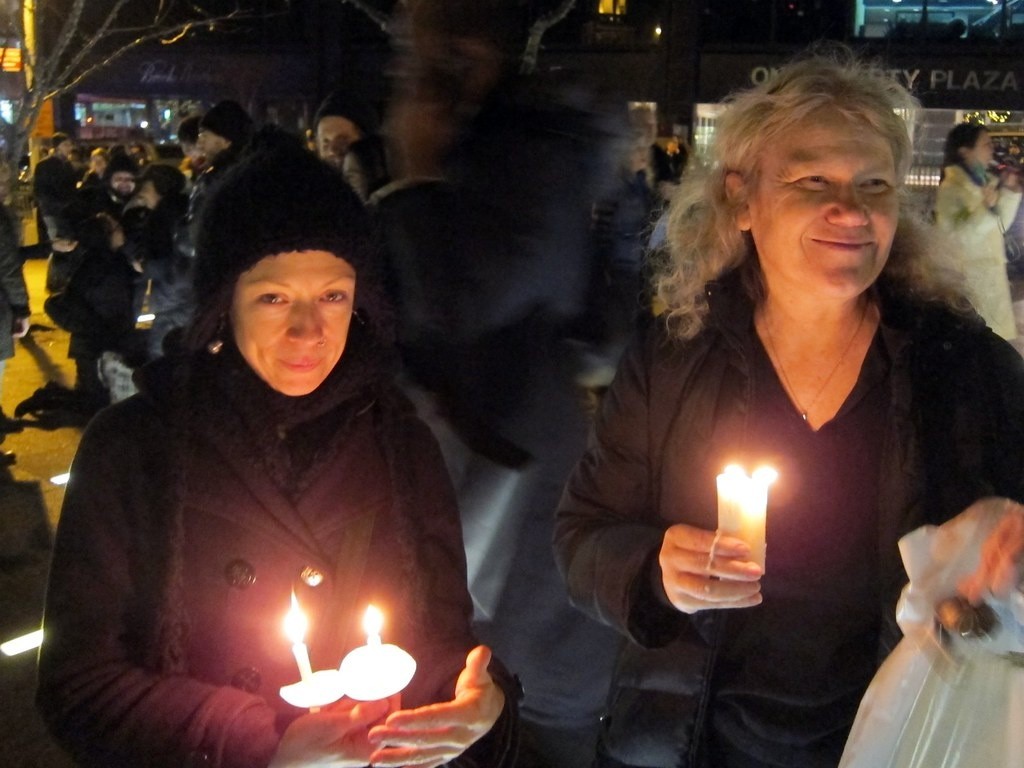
[1,449,18,469]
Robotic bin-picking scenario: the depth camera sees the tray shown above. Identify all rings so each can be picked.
[960,617,975,639]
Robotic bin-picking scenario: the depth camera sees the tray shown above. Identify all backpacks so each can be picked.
[557,129,667,388]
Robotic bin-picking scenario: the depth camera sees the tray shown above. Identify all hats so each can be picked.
[312,83,381,135]
[51,132,69,147]
[201,100,252,141]
[103,146,139,182]
[193,123,375,310]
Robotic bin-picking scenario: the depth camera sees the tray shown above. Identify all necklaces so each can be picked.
[758,294,875,420]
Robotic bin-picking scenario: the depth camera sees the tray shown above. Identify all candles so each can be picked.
[276,588,342,714]
[339,600,417,704]
[714,459,777,578]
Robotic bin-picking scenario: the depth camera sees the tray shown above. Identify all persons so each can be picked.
[552,45,1023,767]
[0,87,711,436]
[22,120,533,768]
[930,122,1022,344]
[322,1,621,742]
